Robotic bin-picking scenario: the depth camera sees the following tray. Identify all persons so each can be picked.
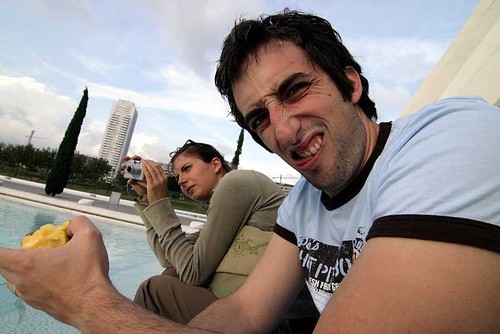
[0,10,500,334]
[118,139,288,323]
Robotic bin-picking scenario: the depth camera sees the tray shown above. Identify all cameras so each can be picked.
[122,160,146,181]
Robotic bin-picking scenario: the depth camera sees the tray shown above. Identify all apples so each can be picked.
[21,218,72,249]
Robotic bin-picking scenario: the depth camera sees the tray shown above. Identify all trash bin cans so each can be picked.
[107,192,122,210]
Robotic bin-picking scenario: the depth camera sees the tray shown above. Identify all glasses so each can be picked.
[170,140,203,163]
